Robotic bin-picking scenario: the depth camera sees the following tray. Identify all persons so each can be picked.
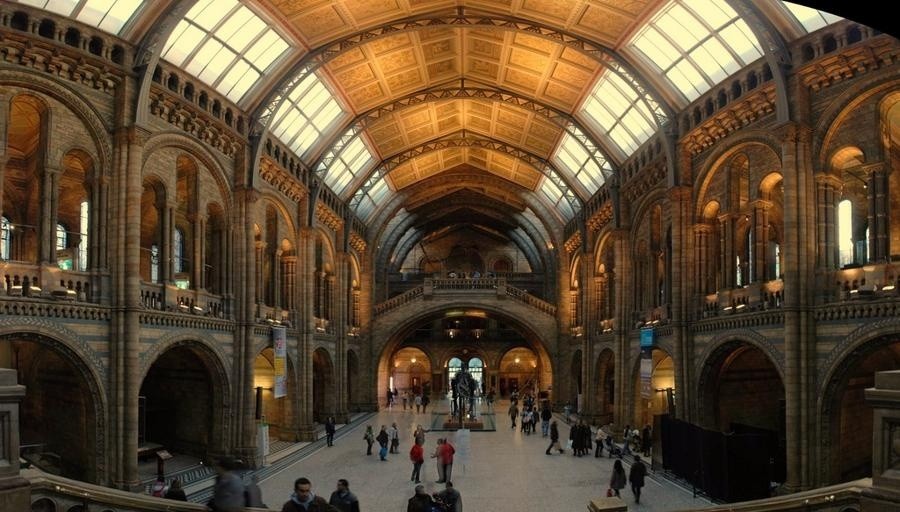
[407,484,441,512]
[434,481,462,512]
[166,480,188,502]
[610,459,627,499]
[628,455,646,504]
[325,417,336,447]
[150,476,169,499]
[481,380,653,457]
[328,479,360,512]
[448,271,498,290]
[281,477,328,512]
[362,387,455,483]
